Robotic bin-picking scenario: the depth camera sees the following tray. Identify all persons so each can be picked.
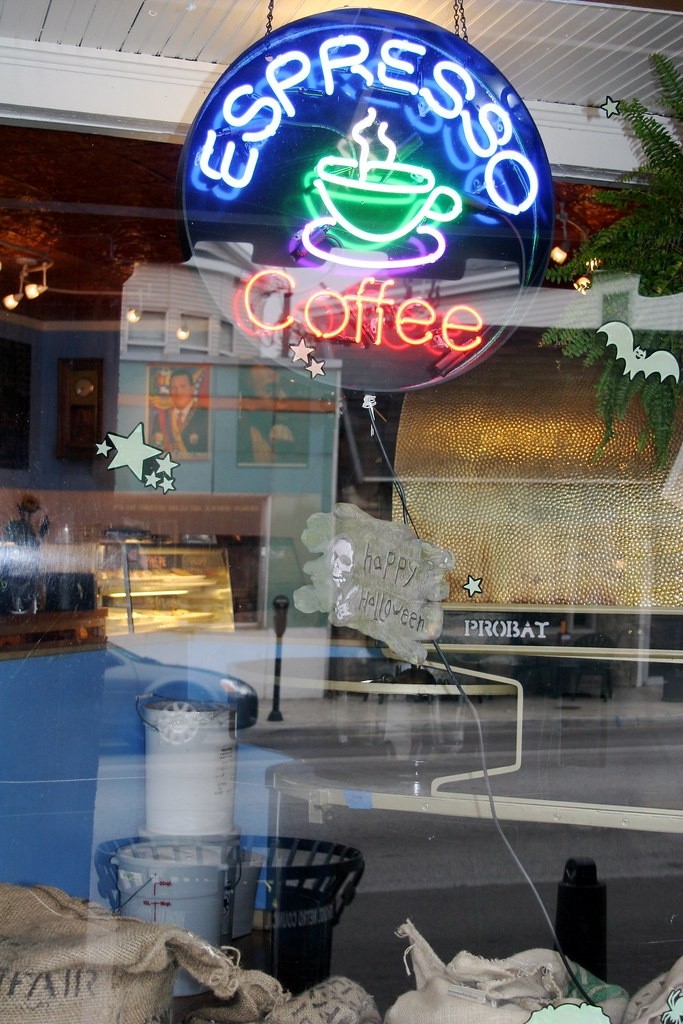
[237,357,310,463]
[151,369,209,453]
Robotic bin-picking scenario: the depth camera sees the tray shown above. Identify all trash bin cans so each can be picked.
[93,835,366,997]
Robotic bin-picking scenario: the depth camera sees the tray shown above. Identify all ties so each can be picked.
[177,413,184,428]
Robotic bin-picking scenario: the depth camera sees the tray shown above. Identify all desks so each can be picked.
[264,743,683,977]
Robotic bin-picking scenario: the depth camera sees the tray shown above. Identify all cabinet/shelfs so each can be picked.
[98,538,237,635]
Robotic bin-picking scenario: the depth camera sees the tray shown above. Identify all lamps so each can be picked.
[125,279,152,324]
[175,323,190,341]
[549,201,603,296]
[2,256,54,312]
